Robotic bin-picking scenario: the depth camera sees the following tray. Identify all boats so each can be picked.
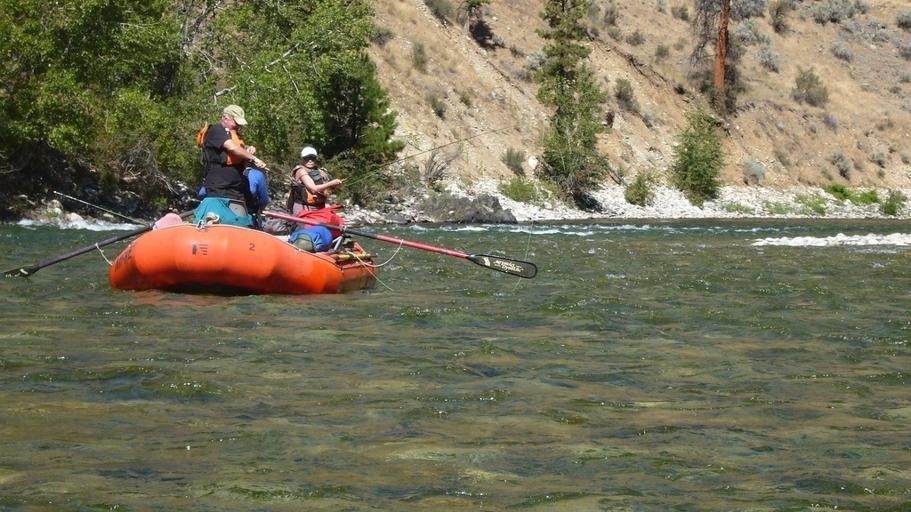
[107,212,375,297]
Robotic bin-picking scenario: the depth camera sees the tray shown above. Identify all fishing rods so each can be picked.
[251,159,395,227]
[53,191,149,226]
[344,127,515,180]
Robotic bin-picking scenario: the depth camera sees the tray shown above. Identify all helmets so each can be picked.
[224,105,248,125]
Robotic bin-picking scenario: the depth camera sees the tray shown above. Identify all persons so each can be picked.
[202,105,267,212]
[291,146,343,230]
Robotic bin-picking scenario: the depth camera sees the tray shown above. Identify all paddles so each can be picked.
[262,210,537,278]
[2,210,196,279]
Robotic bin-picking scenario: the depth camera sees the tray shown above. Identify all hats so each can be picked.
[300,147,317,157]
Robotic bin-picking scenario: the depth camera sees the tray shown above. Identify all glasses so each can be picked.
[305,155,316,160]
